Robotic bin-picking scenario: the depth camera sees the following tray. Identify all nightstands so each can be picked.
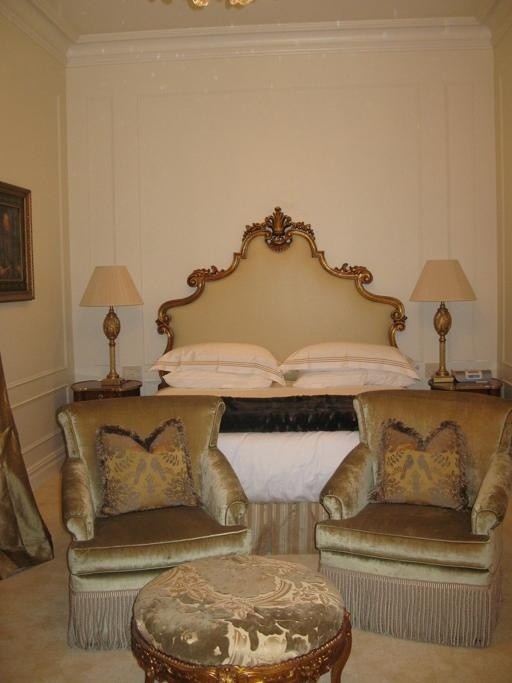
[425,368,505,398]
[69,374,141,403]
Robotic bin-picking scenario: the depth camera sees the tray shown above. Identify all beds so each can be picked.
[154,205,431,555]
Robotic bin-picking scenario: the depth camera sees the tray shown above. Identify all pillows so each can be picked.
[292,369,417,389]
[376,412,470,511]
[160,372,273,391]
[276,340,422,382]
[90,417,202,516]
[149,342,287,391]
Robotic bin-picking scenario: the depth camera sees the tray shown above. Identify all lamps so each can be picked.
[76,263,148,387]
[409,256,479,382]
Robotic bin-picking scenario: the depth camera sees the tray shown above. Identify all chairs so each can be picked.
[313,392,511,647]
[49,394,255,651]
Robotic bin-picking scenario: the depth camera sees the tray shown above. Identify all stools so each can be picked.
[127,555,354,683]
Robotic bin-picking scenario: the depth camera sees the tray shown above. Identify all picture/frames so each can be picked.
[0,179,36,303]
